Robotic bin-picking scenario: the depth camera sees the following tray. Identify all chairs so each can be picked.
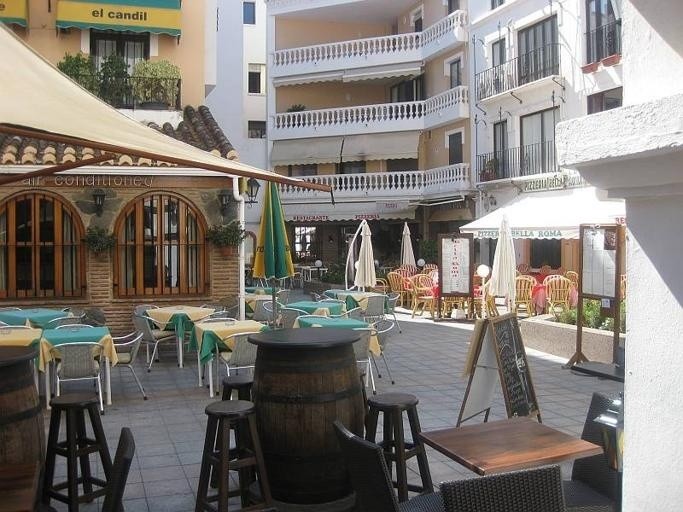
[386,263,499,322]
[101,425,137,512]
[517,262,532,276]
[46,312,87,330]
[320,298,347,304]
[2,325,34,332]
[542,276,577,311]
[262,300,285,328]
[386,292,403,334]
[134,303,161,363]
[59,304,72,313]
[1,306,26,312]
[516,274,538,286]
[352,327,379,396]
[562,270,579,291]
[359,294,386,317]
[436,462,566,512]
[539,264,553,276]
[515,278,535,318]
[199,301,227,313]
[94,328,149,406]
[202,317,239,324]
[330,418,448,512]
[370,319,395,385]
[541,274,565,285]
[130,311,182,373]
[47,340,107,417]
[319,293,331,302]
[338,306,364,324]
[181,311,230,367]
[214,331,261,402]
[251,298,277,322]
[276,289,291,306]
[560,391,622,512]
[516,268,523,277]
[279,307,312,329]
[52,323,96,330]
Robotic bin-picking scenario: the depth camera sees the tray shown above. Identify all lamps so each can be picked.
[244,177,262,209]
[217,188,234,217]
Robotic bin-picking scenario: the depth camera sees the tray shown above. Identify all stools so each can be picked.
[195,399,276,512]
[208,373,253,490]
[363,392,434,504]
[33,389,128,512]
